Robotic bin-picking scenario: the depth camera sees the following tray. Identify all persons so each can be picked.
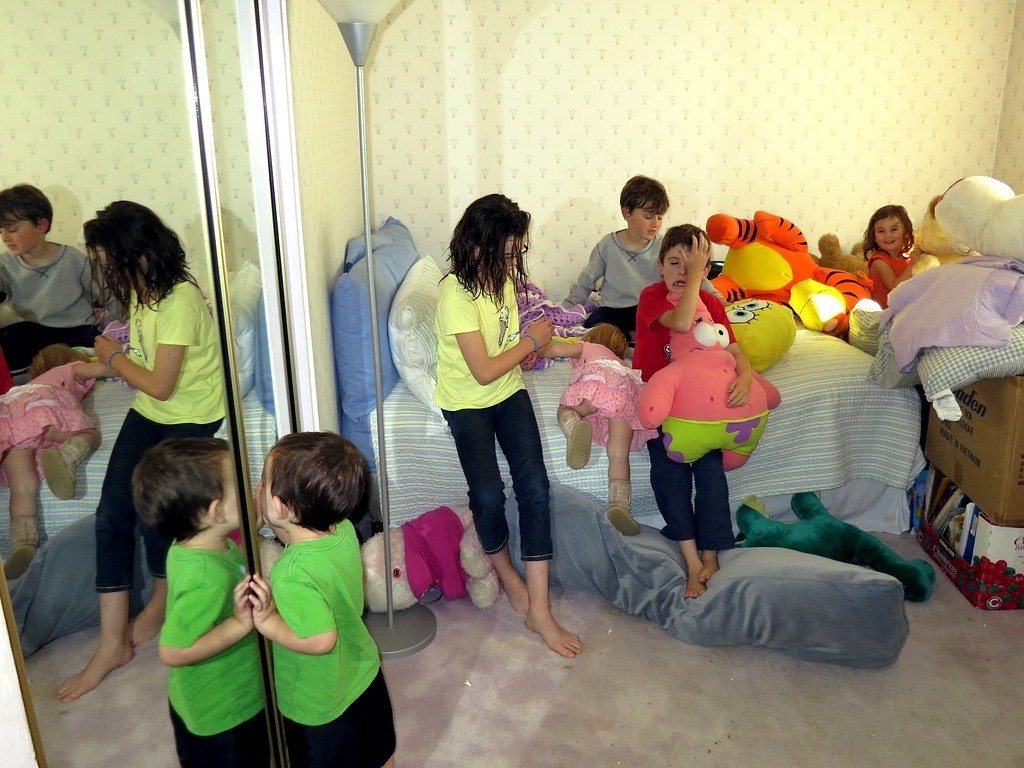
[520,323,659,536]
[249,431,396,768]
[132,436,273,768]
[560,175,732,348]
[0,183,227,704]
[633,223,751,598]
[433,194,583,659]
[863,205,922,310]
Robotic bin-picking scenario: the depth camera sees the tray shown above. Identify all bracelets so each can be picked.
[521,332,539,352]
[107,350,126,369]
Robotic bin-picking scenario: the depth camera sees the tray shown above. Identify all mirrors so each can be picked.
[0,3,300,768]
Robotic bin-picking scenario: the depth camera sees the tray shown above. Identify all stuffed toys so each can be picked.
[723,298,797,373]
[225,527,284,578]
[912,175,1024,276]
[809,233,876,277]
[637,293,781,471]
[361,506,500,614]
[706,210,874,336]
[735,492,936,601]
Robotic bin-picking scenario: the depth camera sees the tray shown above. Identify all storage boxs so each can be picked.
[919,522,1024,611]
[924,375,1024,527]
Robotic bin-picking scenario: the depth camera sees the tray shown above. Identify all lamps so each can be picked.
[313,1,438,657]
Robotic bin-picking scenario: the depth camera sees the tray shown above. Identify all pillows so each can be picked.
[343,217,416,270]
[332,238,422,424]
[397,255,447,422]
[847,307,1024,402]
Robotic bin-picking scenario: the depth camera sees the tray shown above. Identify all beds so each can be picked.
[0,366,228,559]
[334,218,919,542]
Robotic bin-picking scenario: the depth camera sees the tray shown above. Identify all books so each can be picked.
[910,462,982,565]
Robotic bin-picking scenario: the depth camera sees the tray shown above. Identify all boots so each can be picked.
[605,479,641,536]
[558,410,592,471]
[4,515,39,581]
[39,435,92,501]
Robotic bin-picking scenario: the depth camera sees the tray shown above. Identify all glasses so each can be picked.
[505,244,528,260]
[92,258,113,273]
